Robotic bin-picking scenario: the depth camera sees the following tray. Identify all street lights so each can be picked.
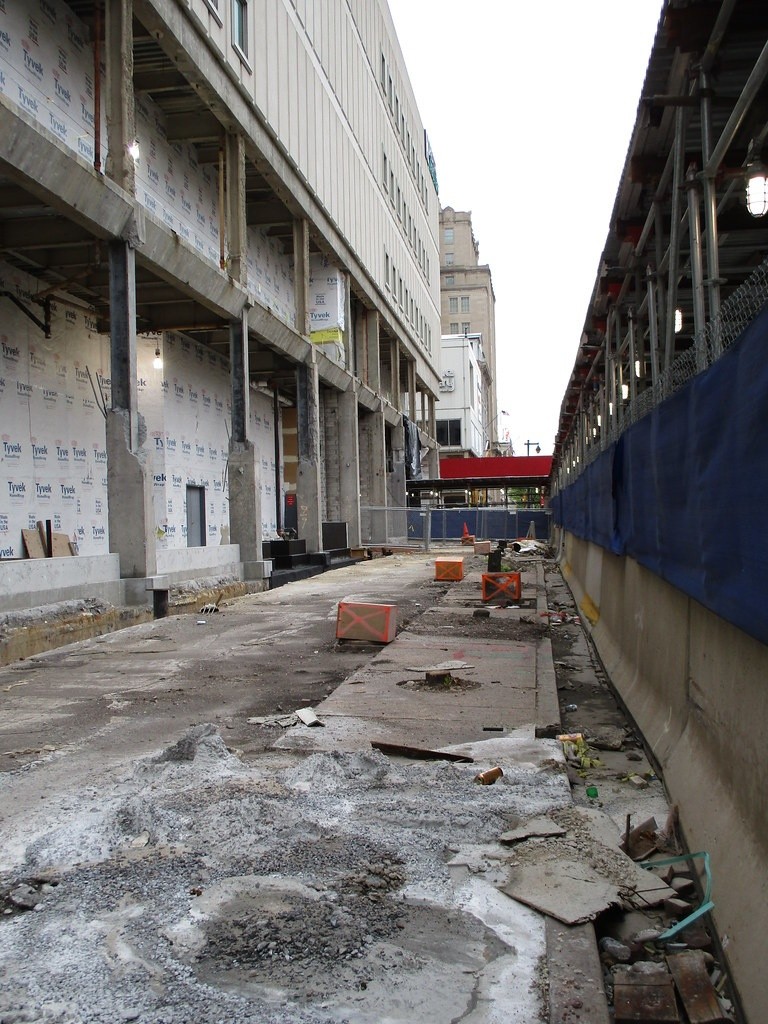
[524,439,542,508]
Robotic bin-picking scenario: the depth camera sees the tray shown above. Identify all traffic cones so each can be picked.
[463,522,469,537]
